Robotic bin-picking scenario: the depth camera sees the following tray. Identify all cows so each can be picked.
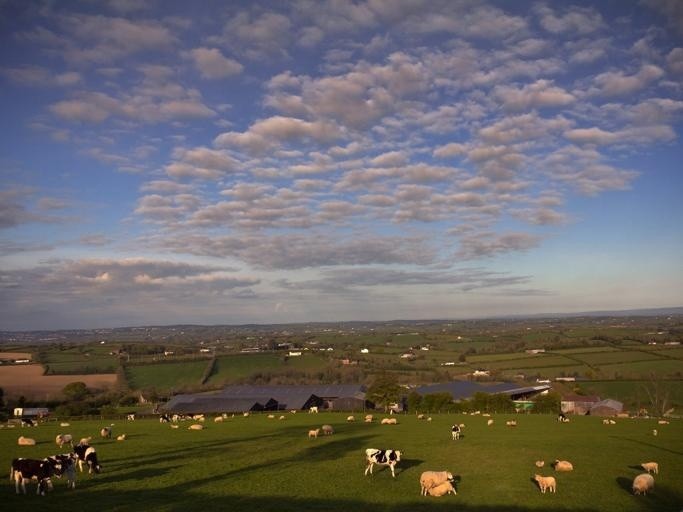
[9,452,78,498]
[73,444,103,475]
[20,418,34,427]
[450,424,461,441]
[364,448,404,478]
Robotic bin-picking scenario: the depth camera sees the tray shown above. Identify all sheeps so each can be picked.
[553,459,573,472]
[127,414,135,422]
[534,474,556,494]
[56,434,74,448]
[602,418,616,425]
[536,460,545,467]
[77,437,92,447]
[322,424,334,436]
[100,426,113,438]
[17,436,37,446]
[346,414,398,424]
[117,434,125,440]
[631,462,659,495]
[417,469,459,497]
[308,429,321,438]
[157,406,319,431]
[557,414,570,423]
